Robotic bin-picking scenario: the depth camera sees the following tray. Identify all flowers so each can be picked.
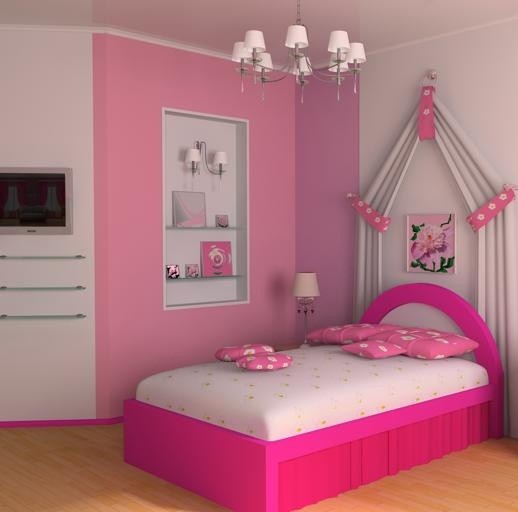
[410,214,454,274]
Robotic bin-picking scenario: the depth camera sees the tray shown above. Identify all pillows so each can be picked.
[367,326,479,360]
[342,340,406,360]
[214,342,274,361]
[306,321,398,344]
[236,351,292,374]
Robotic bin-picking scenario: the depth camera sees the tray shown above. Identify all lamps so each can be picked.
[291,272,320,347]
[229,0,367,108]
[184,137,227,180]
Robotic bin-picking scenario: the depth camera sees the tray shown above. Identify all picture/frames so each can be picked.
[171,190,207,228]
[404,213,457,275]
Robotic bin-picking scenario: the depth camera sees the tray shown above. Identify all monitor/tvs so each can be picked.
[0,166,73,235]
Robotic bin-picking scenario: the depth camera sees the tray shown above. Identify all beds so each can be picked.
[124,283,506,512]
